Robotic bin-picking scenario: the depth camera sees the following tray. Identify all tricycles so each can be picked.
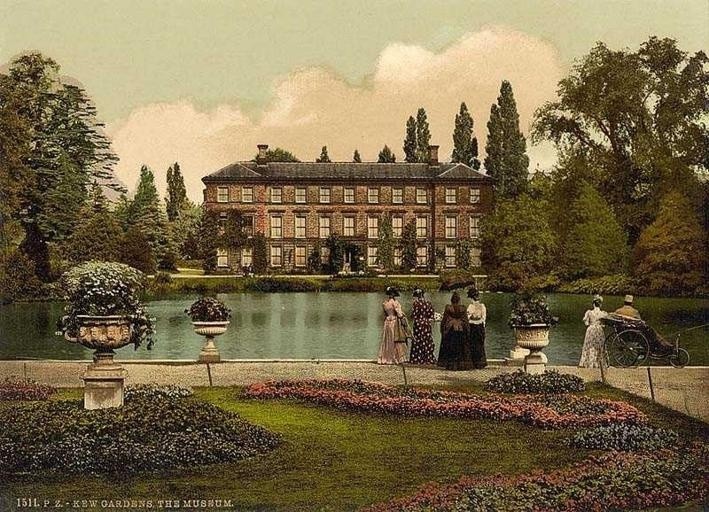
[598,317,691,370]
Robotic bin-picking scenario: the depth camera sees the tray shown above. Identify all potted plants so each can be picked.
[183,297,232,363]
[507,294,559,365]
[54,259,158,411]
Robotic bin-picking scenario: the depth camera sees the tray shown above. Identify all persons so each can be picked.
[465,288,487,369]
[614,295,673,349]
[243,262,255,278]
[436,292,473,371]
[578,295,609,369]
[408,289,437,366]
[377,286,409,365]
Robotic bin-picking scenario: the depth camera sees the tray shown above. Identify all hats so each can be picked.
[624,295,633,303]
